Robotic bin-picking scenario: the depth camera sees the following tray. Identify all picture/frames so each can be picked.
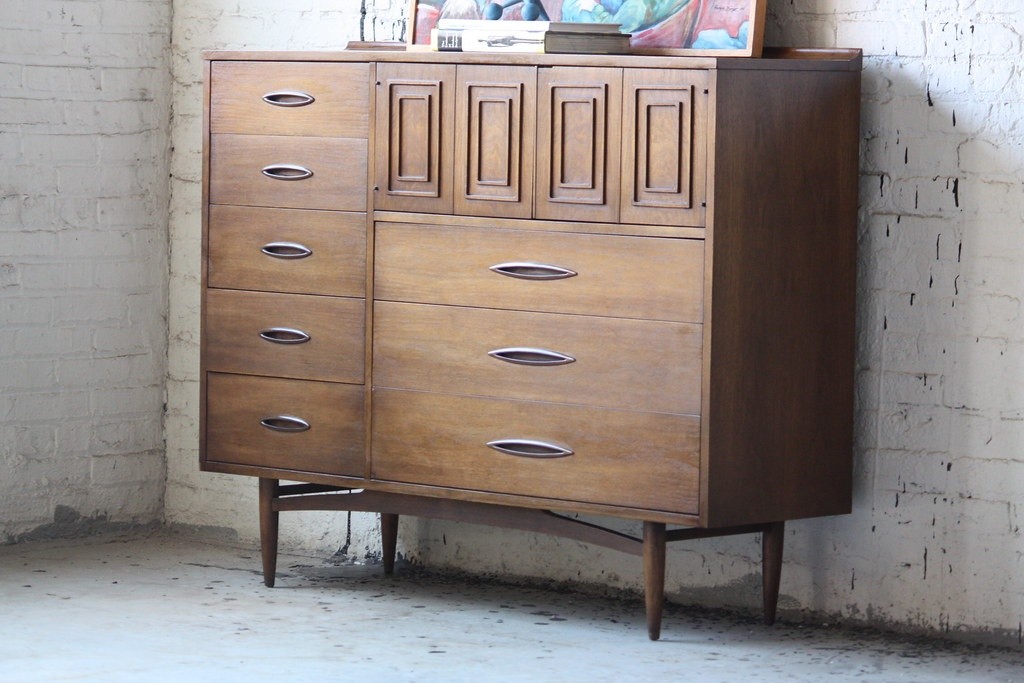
[406,0,766,58]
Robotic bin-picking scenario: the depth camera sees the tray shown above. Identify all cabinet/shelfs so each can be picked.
[196,47,863,642]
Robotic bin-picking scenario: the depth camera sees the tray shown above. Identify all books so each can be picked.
[429,19,634,54]
